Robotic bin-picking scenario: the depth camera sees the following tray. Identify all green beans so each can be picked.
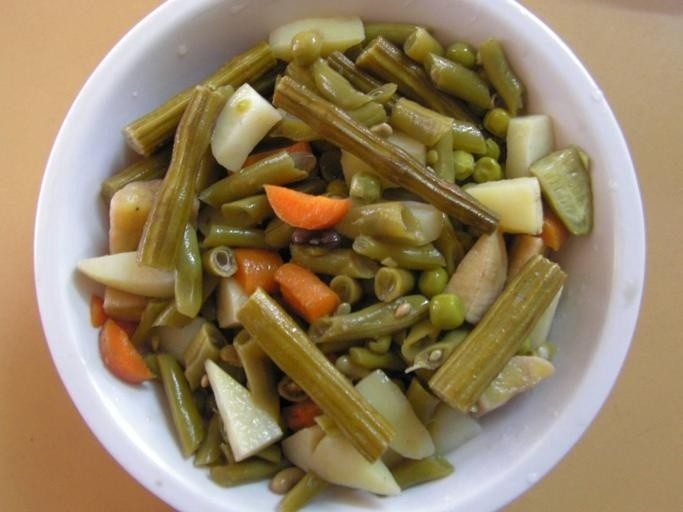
[133,22,551,511]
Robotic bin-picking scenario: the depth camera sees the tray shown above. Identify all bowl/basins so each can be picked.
[34,0,648,512]
